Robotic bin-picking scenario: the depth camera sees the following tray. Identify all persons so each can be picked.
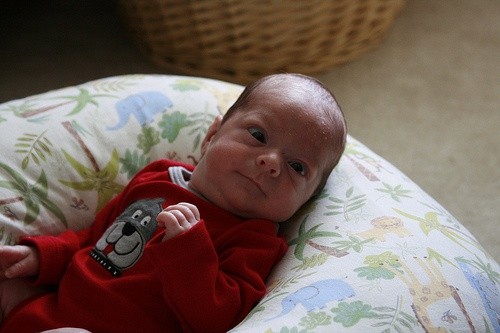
[0,72,349,333]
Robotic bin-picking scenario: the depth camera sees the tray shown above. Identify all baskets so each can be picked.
[120,0,409,85]
